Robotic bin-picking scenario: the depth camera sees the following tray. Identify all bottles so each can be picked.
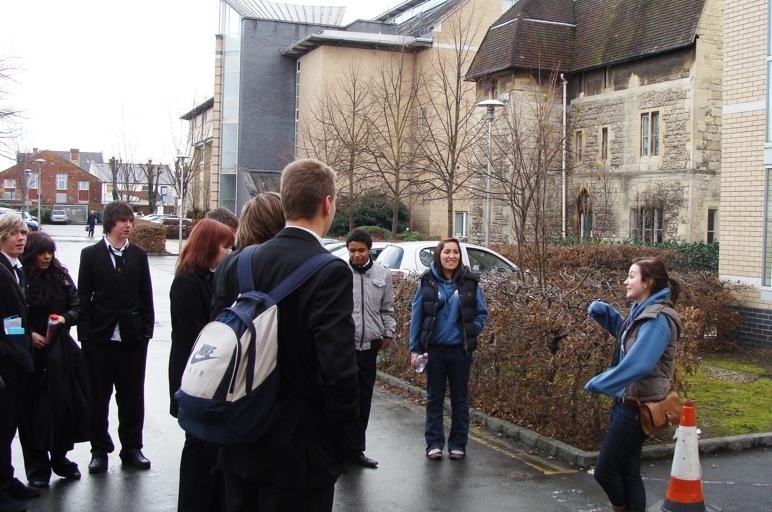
[414,352,429,375]
[45,314,58,344]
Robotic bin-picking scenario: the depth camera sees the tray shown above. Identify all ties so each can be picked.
[109,246,125,270]
[15,265,26,298]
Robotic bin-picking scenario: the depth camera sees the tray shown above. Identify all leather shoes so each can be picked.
[120,449,150,469]
[12,484,41,498]
[89,457,108,472]
[51,460,80,479]
[450,450,464,460]
[353,453,379,467]
[428,449,443,459]
[1,495,27,512]
[32,478,49,487]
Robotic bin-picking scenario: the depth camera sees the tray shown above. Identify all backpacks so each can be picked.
[177,246,348,445]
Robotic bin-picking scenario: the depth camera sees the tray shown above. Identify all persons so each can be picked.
[20,230,82,481]
[0,212,43,512]
[584,254,684,512]
[78,201,154,472]
[169,158,396,511]
[409,238,486,460]
[88,210,97,236]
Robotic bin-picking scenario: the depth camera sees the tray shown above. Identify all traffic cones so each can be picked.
[661,399,705,512]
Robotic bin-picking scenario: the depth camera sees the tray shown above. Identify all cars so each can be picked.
[324,239,538,284]
[50,210,68,223]
[140,213,192,224]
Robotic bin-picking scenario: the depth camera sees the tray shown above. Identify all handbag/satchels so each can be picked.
[641,393,682,435]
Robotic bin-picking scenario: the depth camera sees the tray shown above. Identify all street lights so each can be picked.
[476,100,505,247]
[176,155,190,251]
[24,159,46,224]
